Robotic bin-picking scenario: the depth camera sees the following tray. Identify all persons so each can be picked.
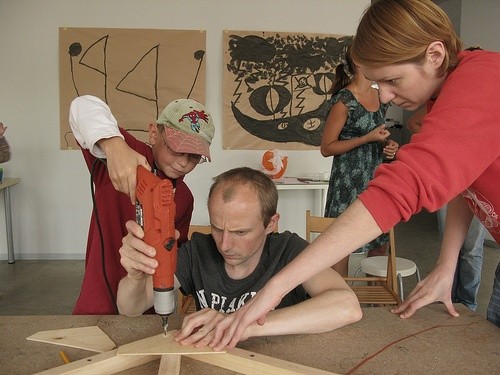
[205,0,500,354]
[69,94,216,317]
[405,43,489,314]
[115,167,363,353]
[319,43,402,309]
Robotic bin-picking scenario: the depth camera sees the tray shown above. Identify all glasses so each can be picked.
[161,132,207,164]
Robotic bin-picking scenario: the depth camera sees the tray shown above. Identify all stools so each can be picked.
[352,256,421,286]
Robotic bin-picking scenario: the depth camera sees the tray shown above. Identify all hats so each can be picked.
[156,98,215,163]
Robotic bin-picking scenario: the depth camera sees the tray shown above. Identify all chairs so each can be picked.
[306,210,402,306]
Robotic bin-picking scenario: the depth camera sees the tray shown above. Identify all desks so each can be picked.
[0,177,21,264]
[0,303,500,375]
[274,177,329,217]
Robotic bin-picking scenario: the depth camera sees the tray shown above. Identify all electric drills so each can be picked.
[131,165,177,335]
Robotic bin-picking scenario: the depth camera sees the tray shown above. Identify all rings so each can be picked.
[393,152,396,156]
